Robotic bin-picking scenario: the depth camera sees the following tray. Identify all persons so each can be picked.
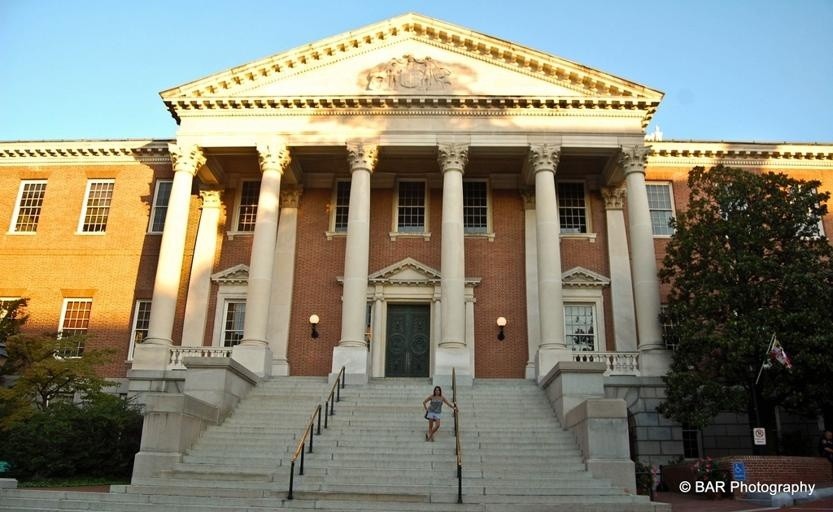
[423,386,458,441]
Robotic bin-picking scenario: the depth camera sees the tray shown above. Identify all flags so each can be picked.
[769,337,793,375]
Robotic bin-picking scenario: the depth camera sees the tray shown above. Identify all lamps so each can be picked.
[310,314,319,339]
[497,317,507,340]
